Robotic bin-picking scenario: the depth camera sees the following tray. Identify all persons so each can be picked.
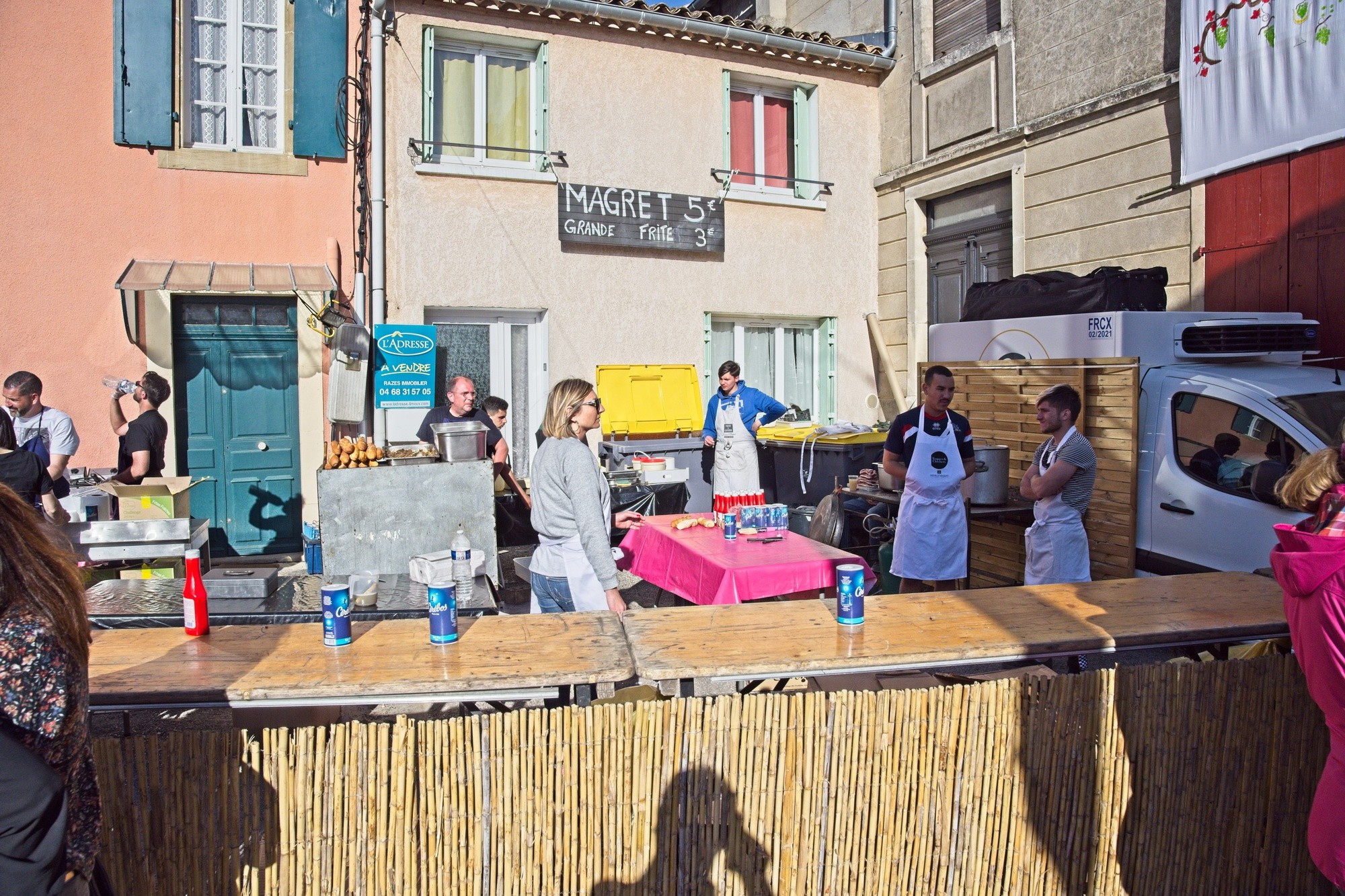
[416,376,531,547]
[102,371,171,520]
[1269,417,1345,896]
[701,360,788,512]
[1189,433,1295,494]
[528,374,646,769]
[1019,380,1097,674]
[839,424,905,573]
[0,371,103,895]
[882,365,976,594]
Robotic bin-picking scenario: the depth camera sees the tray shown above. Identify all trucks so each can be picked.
[928,310,1345,653]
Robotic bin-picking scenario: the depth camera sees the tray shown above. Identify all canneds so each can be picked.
[740,503,789,529]
[724,513,737,540]
[428,582,458,646]
[836,564,865,626]
[320,583,352,647]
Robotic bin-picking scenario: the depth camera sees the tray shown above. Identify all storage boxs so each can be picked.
[120,559,186,580]
[301,521,323,574]
[96,476,210,520]
[625,456,689,486]
[198,567,279,599]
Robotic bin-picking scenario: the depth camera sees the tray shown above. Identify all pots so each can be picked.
[960,439,1010,504]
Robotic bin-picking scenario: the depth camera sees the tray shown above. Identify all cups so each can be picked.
[321,583,352,647]
[848,475,858,491]
[428,582,458,645]
[723,503,788,541]
[836,564,865,626]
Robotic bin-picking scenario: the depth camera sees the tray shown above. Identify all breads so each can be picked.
[325,436,384,470]
[671,516,715,530]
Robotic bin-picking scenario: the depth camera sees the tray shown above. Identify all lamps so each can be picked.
[318,308,346,328]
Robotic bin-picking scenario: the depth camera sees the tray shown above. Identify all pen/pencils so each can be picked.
[747,537,783,544]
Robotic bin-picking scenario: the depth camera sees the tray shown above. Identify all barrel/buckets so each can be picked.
[631,450,675,471]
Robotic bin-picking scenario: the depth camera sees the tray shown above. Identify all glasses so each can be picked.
[581,398,601,411]
[135,381,144,390]
[451,391,477,398]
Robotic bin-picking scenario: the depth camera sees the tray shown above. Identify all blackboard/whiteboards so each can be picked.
[558,182,725,254]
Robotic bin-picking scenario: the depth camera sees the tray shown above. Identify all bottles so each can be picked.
[713,489,765,530]
[102,375,138,394]
[451,530,472,600]
[183,549,210,635]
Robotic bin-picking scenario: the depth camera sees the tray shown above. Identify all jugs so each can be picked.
[348,569,380,606]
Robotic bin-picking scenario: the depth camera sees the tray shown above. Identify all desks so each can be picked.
[79,573,498,628]
[613,511,877,605]
[87,571,1292,741]
[835,477,1035,588]
[70,519,212,576]
